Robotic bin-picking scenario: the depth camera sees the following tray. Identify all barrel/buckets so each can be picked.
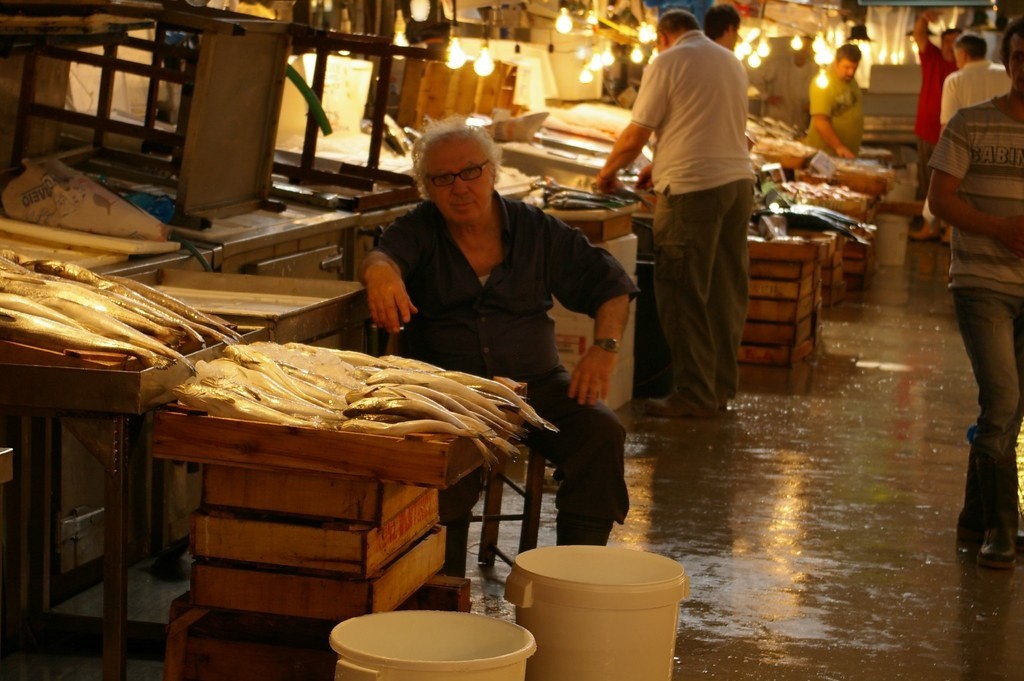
[874,215,911,265]
[329,610,537,681]
[505,545,690,681]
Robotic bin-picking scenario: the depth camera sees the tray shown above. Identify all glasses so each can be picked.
[425,159,490,187]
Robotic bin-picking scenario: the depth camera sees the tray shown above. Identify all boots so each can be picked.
[956,442,1024,550]
[975,453,1018,569]
[908,216,940,240]
[942,225,951,244]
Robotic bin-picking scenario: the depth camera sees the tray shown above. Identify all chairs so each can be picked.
[368,224,545,573]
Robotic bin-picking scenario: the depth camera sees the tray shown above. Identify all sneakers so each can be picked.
[643,390,727,416]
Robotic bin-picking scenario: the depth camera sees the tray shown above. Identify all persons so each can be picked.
[906,8,1011,244]
[762,35,864,155]
[926,18,1024,568]
[356,111,630,575]
[590,6,756,420]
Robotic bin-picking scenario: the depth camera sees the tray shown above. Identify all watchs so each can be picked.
[593,338,620,352]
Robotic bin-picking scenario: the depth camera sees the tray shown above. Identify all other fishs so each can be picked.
[0,248,247,378]
[751,204,877,246]
[171,341,560,472]
[530,174,654,214]
